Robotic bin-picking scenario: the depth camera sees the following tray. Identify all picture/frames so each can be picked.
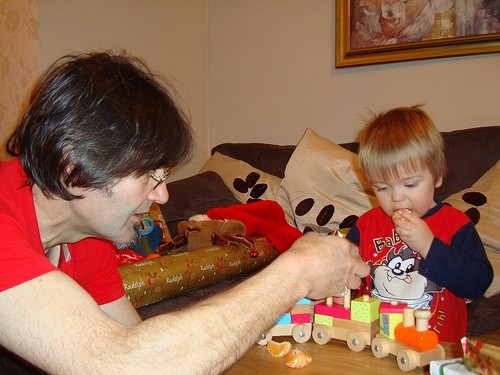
[335,0,500,69]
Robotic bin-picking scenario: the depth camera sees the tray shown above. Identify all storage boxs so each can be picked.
[457,331,500,375]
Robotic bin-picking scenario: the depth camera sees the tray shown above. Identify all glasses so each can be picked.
[146,170,171,191]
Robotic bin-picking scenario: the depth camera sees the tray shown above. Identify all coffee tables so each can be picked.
[217,328,500,375]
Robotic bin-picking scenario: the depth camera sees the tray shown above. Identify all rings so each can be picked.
[340,285,348,297]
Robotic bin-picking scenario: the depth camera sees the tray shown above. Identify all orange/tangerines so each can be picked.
[285,348,312,368]
[392,208,412,224]
[267,340,292,357]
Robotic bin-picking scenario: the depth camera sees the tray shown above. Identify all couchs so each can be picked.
[138,126,500,341]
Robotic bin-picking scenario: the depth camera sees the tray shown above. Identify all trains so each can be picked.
[256,294,445,372]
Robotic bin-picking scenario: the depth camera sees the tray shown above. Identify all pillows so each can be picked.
[439,157,500,300]
[274,127,379,238]
[198,150,282,204]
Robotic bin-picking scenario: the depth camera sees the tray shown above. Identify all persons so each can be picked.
[0,50,371,375]
[346,104,494,339]
